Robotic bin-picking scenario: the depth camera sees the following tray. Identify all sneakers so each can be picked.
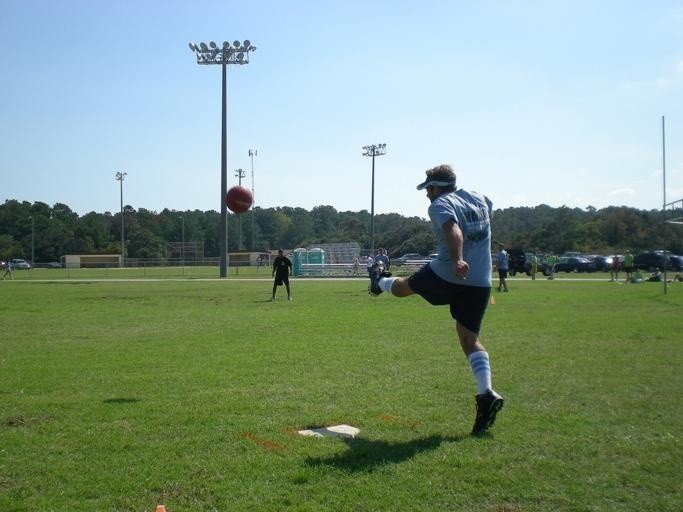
[495,288,508,292]
[368,261,392,296]
[271,297,293,301]
[471,389,504,437]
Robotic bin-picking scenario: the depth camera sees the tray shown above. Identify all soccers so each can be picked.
[227,186,252,213]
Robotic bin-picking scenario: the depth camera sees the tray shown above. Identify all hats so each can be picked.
[417,176,456,190]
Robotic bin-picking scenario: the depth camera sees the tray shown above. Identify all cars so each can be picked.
[507,248,625,276]
[636,251,683,271]
[398,254,438,260]
[10,259,30,268]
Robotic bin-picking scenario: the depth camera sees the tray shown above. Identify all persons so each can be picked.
[270,250,292,300]
[256,256,261,272]
[354,260,359,274]
[368,165,504,435]
[495,243,510,292]
[531,251,538,280]
[367,248,390,278]
[610,250,683,284]
[3,259,12,280]
[547,251,556,280]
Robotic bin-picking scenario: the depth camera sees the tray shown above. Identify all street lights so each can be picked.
[189,40,256,277]
[28,216,34,263]
[234,168,247,250]
[116,172,127,267]
[249,149,257,252]
[362,144,386,249]
[179,215,185,260]
